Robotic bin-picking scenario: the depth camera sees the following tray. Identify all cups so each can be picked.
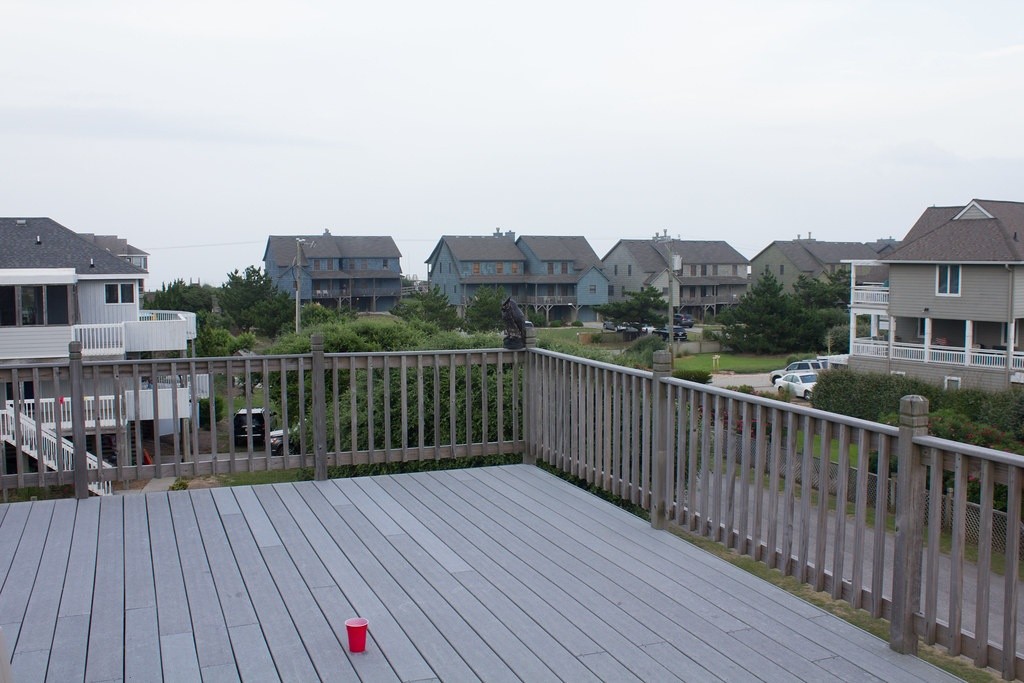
[345,618,369,653]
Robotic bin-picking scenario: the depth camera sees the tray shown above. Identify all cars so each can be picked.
[774,371,821,401]
[673,314,695,328]
[626,324,656,335]
[652,327,687,342]
[603,318,630,332]
[270,419,307,456]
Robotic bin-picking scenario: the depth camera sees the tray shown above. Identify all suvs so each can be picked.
[233,407,278,447]
[769,359,831,386]
